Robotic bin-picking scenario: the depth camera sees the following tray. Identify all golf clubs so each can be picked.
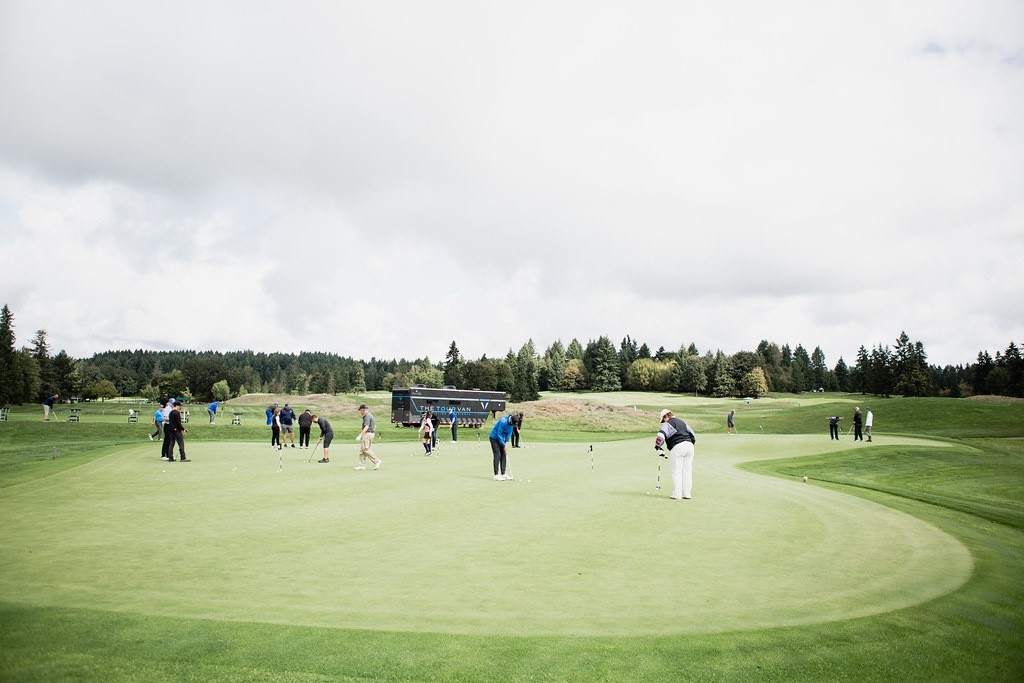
[506,451,515,481]
[414,434,420,455]
[519,433,525,448]
[655,457,661,491]
[847,424,854,436]
[307,440,321,463]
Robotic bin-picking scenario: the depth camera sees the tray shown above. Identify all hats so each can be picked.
[853,407,859,410]
[305,409,311,414]
[660,409,673,423]
[284,402,289,406]
[732,409,736,414]
[169,398,176,404]
[511,415,519,427]
[358,404,369,411]
[173,402,183,407]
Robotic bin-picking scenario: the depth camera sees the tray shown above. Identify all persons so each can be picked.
[44,394,59,420]
[826,416,842,440]
[655,408,696,499]
[728,409,735,435]
[208,401,220,424]
[353,405,381,470]
[417,412,440,456]
[448,408,458,444]
[149,393,192,462]
[298,410,312,449]
[853,406,863,442]
[311,415,334,463]
[266,403,298,449]
[489,413,524,481]
[865,407,873,442]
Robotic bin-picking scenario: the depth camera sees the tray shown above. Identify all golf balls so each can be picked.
[527,480,530,483]
[304,461,305,462]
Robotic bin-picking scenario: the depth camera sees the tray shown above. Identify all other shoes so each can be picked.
[209,422,215,424]
[149,433,153,441]
[450,440,457,444]
[161,456,176,462]
[158,439,163,441]
[670,496,691,499]
[284,444,295,447]
[425,447,434,456]
[512,445,520,449]
[180,459,191,462]
[300,446,309,449]
[271,444,282,449]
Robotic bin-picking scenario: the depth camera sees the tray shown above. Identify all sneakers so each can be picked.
[493,474,512,481]
[353,460,382,470]
[318,458,330,463]
